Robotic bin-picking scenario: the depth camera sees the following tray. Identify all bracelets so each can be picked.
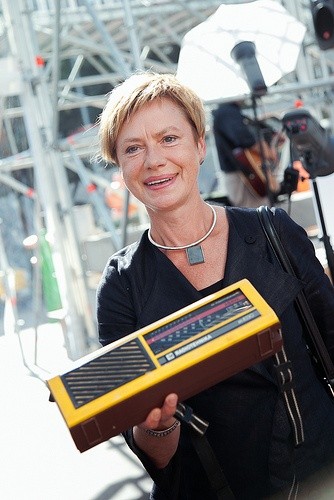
[140,420,181,436]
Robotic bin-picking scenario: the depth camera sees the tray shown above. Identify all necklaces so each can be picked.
[147,202,218,266]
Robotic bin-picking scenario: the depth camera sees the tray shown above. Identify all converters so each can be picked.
[278,166,300,194]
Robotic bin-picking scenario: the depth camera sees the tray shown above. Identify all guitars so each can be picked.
[233,100,303,201]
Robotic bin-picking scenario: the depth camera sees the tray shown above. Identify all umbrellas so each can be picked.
[175,0,308,103]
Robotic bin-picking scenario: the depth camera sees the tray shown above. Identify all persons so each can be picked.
[97,70,334,500]
[210,98,288,206]
